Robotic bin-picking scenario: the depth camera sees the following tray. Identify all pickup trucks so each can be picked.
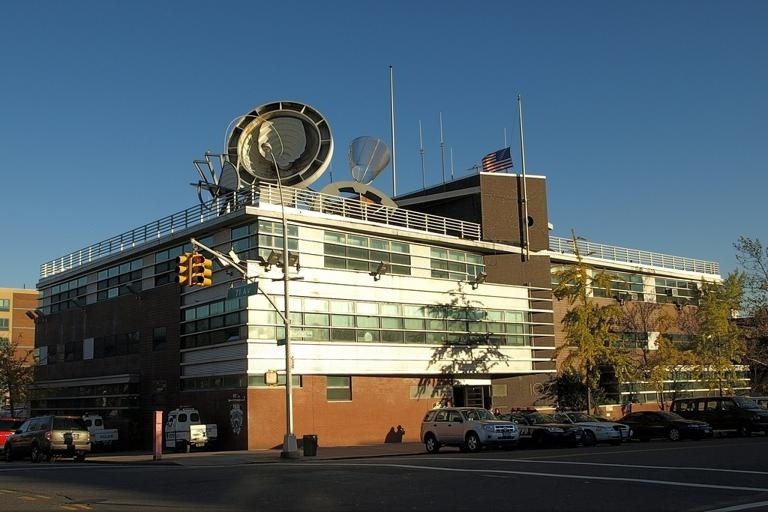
[83,414,119,450]
[165,406,221,452]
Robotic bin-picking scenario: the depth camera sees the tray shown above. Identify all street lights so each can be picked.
[260,141,300,463]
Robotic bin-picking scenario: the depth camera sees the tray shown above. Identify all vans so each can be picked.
[668,394,767,436]
[751,397,767,410]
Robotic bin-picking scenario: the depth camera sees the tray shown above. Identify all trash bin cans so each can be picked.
[304,435,317,456]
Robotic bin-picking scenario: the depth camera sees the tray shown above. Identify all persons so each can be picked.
[381,424,406,442]
[493,407,503,417]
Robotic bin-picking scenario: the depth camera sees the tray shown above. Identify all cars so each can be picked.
[553,412,634,446]
[488,410,584,450]
[615,409,712,441]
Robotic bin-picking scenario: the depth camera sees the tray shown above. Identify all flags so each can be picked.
[481,147,515,173]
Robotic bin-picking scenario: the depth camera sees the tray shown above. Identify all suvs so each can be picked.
[420,406,519,452]
[4,416,92,463]
[0,418,29,455]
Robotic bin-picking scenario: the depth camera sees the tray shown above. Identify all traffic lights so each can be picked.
[175,253,191,287]
[191,254,204,288]
[202,259,214,288]
